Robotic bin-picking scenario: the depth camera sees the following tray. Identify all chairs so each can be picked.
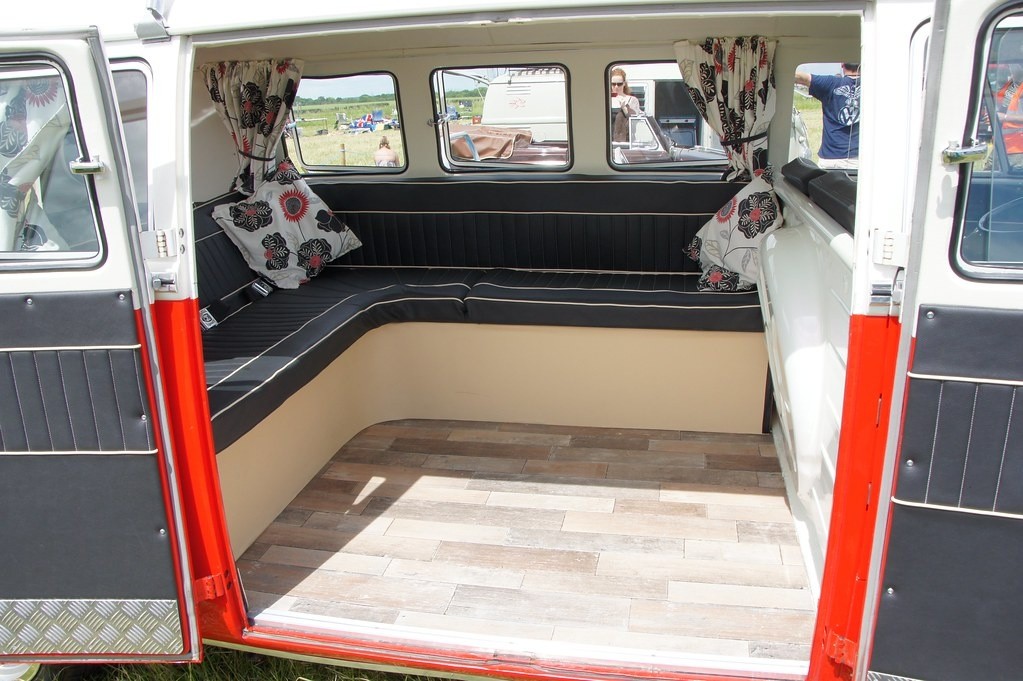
[779,152,857,237]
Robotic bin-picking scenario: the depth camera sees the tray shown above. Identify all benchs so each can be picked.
[204,171,764,589]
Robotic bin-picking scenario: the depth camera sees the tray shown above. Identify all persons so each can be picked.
[374,135,400,167]
[981,64,1023,171]
[610,67,640,142]
[794,63,862,169]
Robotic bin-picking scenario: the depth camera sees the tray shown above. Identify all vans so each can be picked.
[481,61,815,166]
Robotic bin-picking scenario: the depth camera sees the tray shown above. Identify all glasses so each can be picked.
[612,80,625,86]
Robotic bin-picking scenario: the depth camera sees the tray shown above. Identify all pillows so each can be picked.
[684,162,786,293]
[210,153,364,289]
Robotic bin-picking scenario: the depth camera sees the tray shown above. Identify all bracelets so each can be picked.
[1004,114,1007,122]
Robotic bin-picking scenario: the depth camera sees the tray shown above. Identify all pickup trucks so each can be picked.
[449,112,730,164]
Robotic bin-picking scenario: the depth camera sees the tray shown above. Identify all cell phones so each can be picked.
[611,92,618,97]
